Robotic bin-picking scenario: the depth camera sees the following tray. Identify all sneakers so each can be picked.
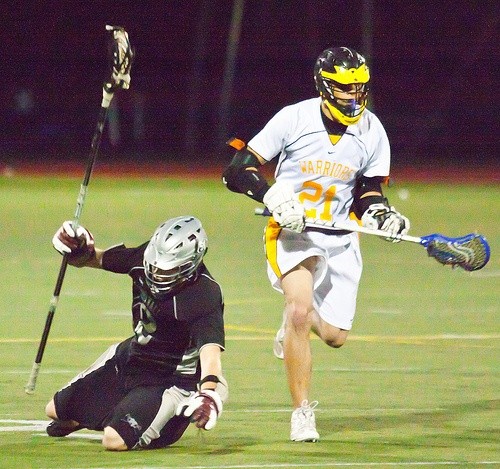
[46,420,84,437]
[273,310,286,359]
[290,400,320,443]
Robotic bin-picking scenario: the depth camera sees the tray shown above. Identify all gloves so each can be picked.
[360,203,410,243]
[52,220,95,269]
[176,389,224,430]
[262,178,306,233]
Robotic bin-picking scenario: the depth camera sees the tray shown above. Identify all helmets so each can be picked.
[142,215,209,299]
[313,46,370,125]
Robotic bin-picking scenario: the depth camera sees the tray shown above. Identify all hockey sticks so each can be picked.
[26,24,136,395]
[254,207,491,272]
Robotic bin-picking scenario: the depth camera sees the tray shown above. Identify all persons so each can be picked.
[44,214,228,450]
[223,46,410,442]
